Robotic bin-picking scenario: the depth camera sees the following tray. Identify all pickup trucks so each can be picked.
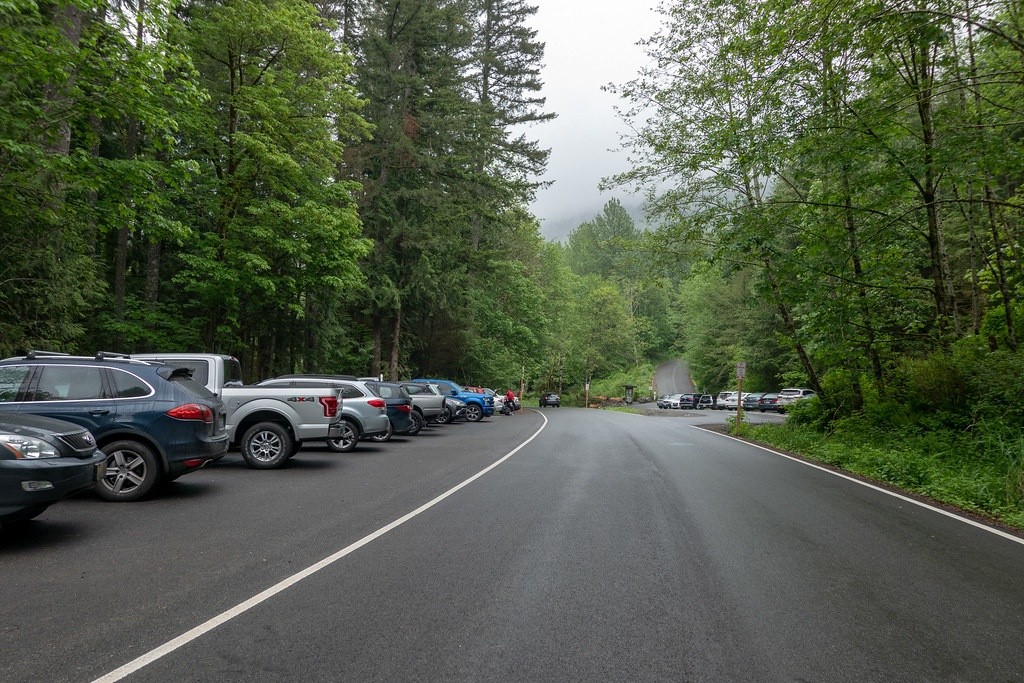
[357,373,446,436]
[115,353,346,471]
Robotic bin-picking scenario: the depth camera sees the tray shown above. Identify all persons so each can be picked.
[505,387,516,414]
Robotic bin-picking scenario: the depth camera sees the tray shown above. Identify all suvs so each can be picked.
[716,391,737,410]
[253,374,388,454]
[777,386,819,415]
[0,351,229,501]
[359,378,414,443]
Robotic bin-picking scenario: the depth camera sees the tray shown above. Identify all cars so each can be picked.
[0,410,107,523]
[656,393,718,410]
[724,392,753,411]
[396,379,521,424]
[757,392,780,413]
[538,392,561,408]
[742,392,768,411]
